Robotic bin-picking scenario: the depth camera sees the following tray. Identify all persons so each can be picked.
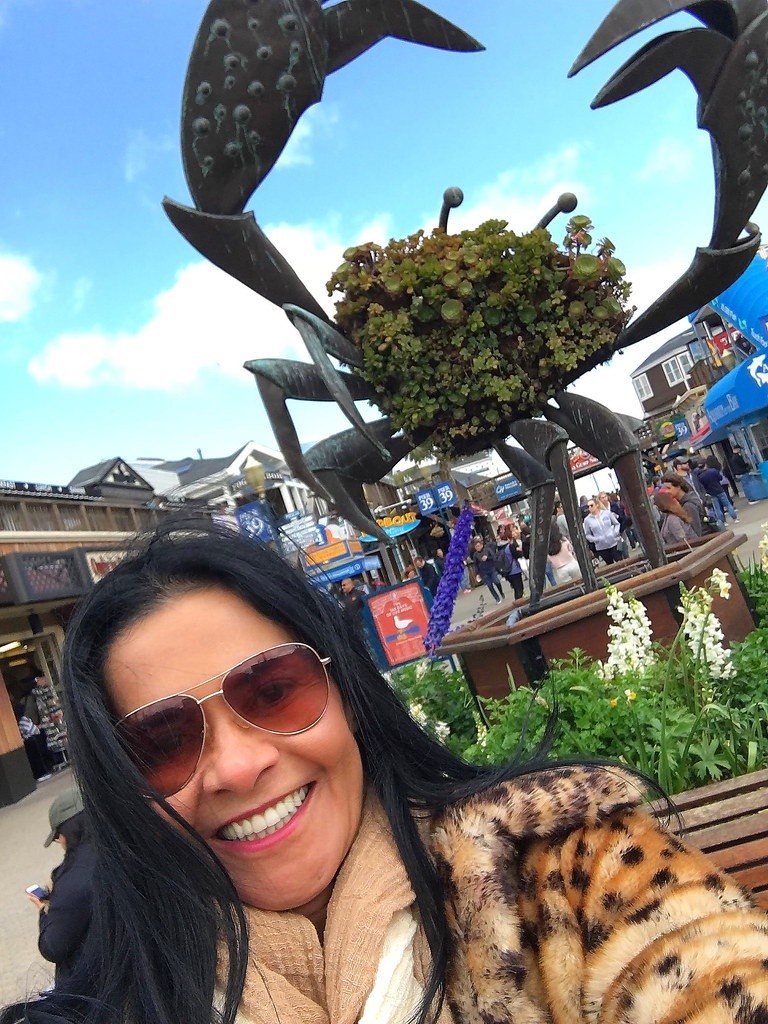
[342,578,386,639]
[646,444,751,546]
[404,549,472,600]
[469,501,580,604]
[1,517,768,1024]
[27,789,92,984]
[579,490,638,566]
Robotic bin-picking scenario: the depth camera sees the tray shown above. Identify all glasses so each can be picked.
[341,584,347,587]
[586,504,593,508]
[109,642,331,799]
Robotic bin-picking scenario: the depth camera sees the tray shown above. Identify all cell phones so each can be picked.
[25,884,46,900]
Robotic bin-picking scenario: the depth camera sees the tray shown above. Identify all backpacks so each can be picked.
[493,543,515,577]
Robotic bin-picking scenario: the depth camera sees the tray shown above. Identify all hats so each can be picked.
[697,460,706,468]
[734,444,744,449]
[672,456,689,467]
[44,788,86,848]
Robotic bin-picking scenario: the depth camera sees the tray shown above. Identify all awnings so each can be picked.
[705,347,768,430]
[689,426,728,449]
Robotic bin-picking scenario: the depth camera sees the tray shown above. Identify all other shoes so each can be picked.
[723,507,741,526]
[748,500,760,505]
